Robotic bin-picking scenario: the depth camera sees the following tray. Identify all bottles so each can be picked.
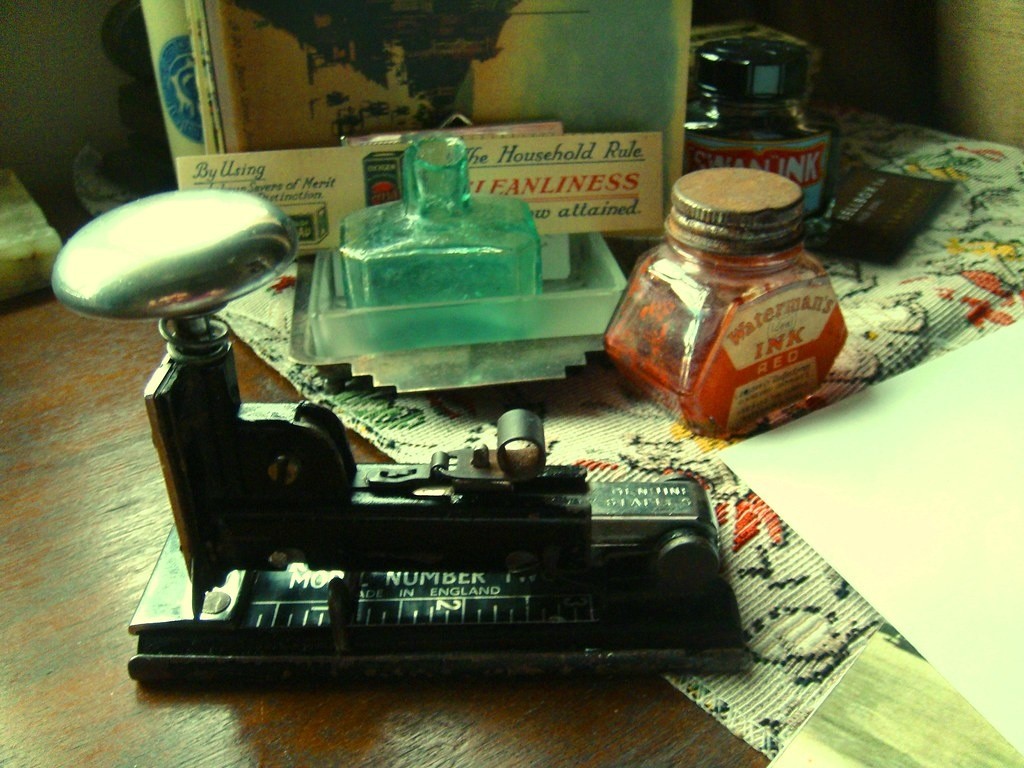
[338,134,543,353]
[682,36,844,252]
[602,168,848,441]
